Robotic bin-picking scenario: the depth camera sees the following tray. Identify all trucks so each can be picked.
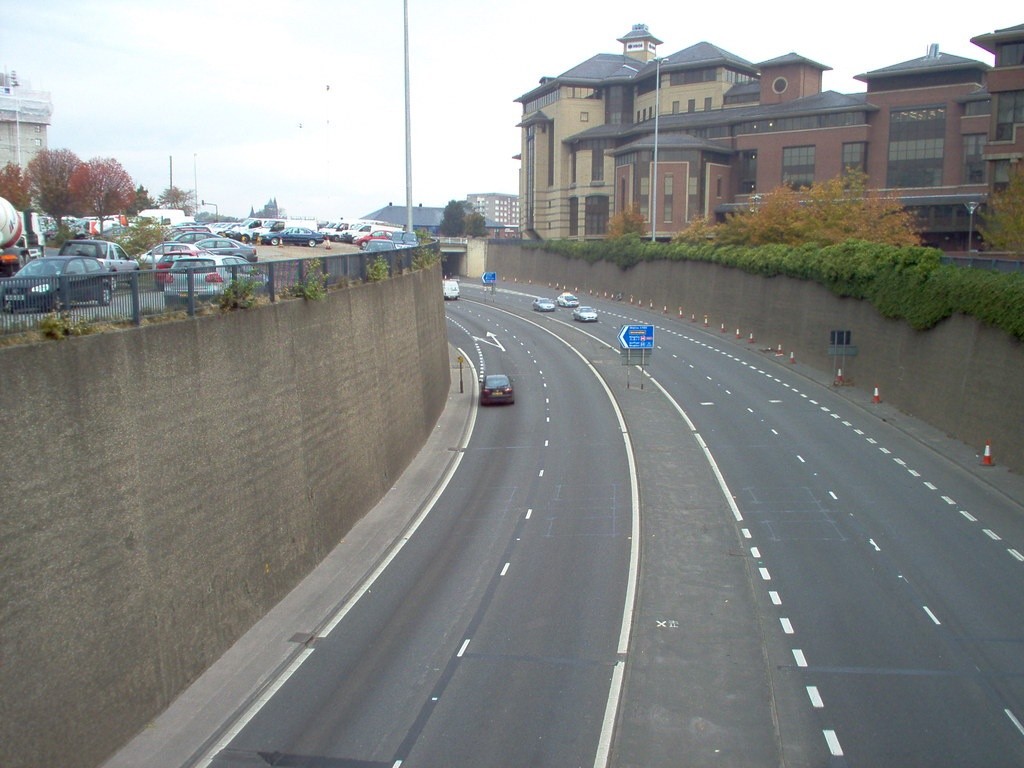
[441,279,460,301]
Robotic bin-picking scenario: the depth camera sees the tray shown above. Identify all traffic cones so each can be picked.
[835,369,844,384]
[502,275,798,366]
[976,440,996,466]
[871,382,884,404]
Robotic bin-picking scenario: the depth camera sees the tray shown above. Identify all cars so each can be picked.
[532,298,556,312]
[573,305,598,322]
[478,374,516,405]
[0,195,421,315]
[557,292,579,307]
